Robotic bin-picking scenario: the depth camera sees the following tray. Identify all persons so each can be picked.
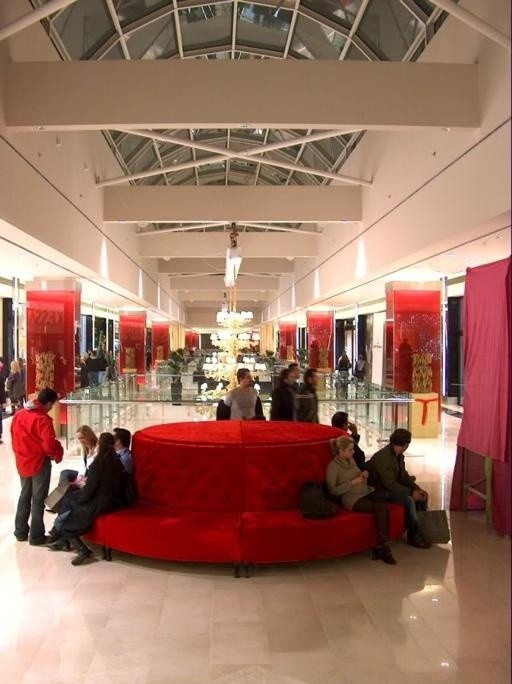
[289,364,300,379]
[338,351,351,377]
[366,428,432,549]
[356,354,368,382]
[217,369,264,421]
[299,368,319,422]
[0,347,138,565]
[270,369,299,421]
[327,437,397,565]
[332,412,366,462]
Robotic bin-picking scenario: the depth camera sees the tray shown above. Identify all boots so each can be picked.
[69,536,94,566]
[50,538,71,550]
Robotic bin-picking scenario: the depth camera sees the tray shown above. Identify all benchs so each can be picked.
[82,420,242,575]
[242,421,406,576]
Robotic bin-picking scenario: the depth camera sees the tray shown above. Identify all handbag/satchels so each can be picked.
[43,481,80,515]
[416,509,450,544]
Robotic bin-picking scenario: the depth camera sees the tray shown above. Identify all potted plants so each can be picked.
[168,348,186,393]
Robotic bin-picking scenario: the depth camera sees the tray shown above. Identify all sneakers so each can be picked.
[407,535,431,549]
[15,533,29,540]
[378,544,396,565]
[30,533,56,545]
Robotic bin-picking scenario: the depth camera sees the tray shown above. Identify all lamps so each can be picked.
[198,222,268,406]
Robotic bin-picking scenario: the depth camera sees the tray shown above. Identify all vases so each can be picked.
[171,393,182,402]
